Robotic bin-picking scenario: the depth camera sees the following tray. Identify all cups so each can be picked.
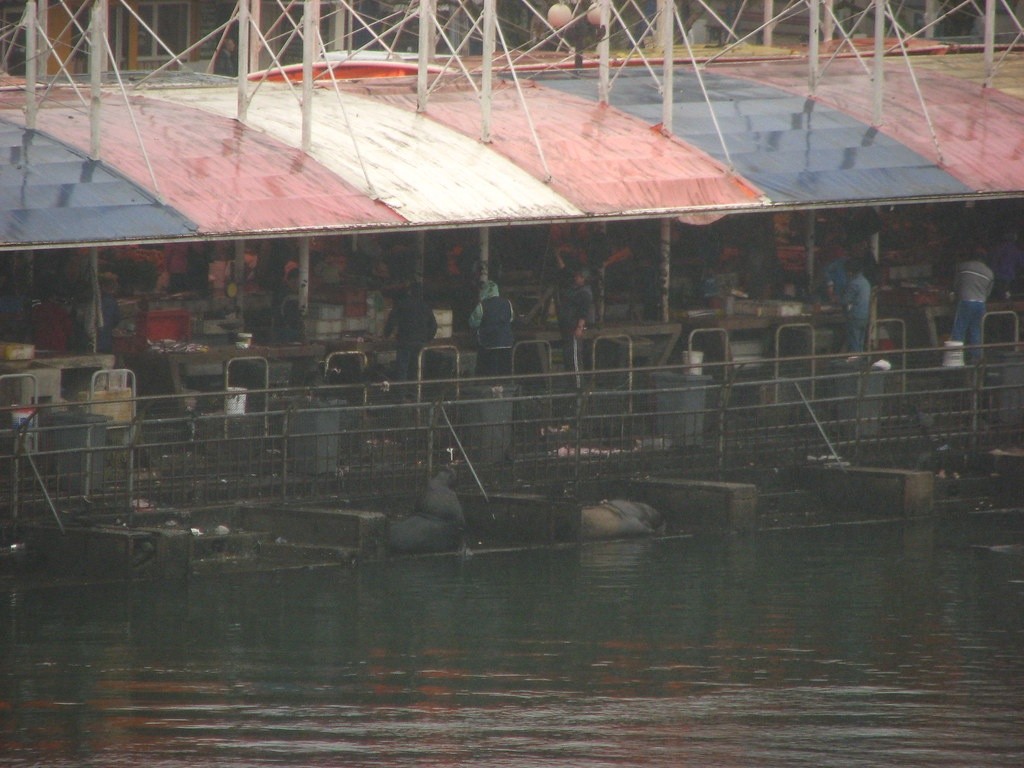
[235,332,253,350]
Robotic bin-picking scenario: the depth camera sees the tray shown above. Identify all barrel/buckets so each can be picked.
[943,340,965,368]
[681,351,704,375]
[224,386,248,415]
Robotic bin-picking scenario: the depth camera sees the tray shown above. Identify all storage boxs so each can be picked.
[0,343,35,359]
[135,307,243,346]
[307,284,452,339]
[737,300,801,316]
[892,291,938,310]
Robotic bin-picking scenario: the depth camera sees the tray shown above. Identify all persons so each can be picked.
[0,196,1024,410]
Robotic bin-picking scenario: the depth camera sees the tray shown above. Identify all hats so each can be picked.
[976,247,987,259]
